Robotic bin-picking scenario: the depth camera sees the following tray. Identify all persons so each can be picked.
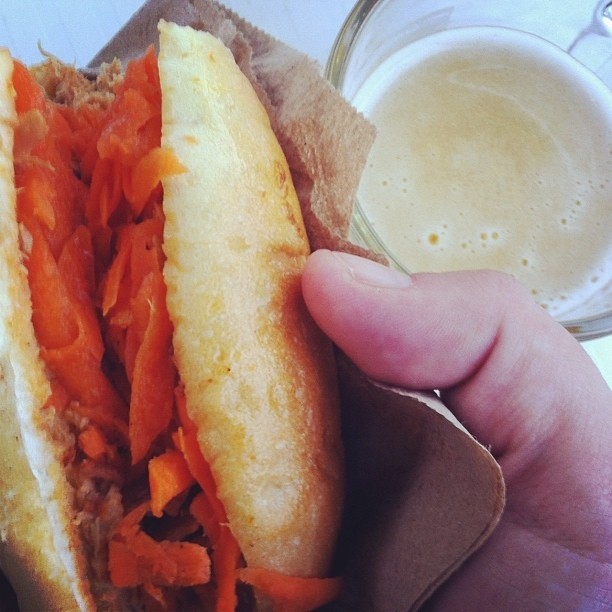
[301,248,612,612]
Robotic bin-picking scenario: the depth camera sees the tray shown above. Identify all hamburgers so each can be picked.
[2,14,351,612]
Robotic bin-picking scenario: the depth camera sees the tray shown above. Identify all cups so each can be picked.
[322,0,612,341]
